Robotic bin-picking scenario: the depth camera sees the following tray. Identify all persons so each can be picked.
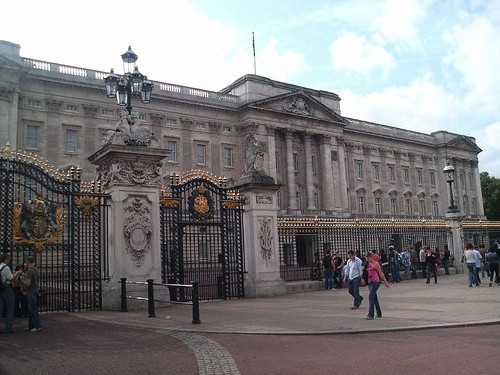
[361,253,390,321]
[323,246,450,290]
[0,254,41,333]
[464,243,500,287]
[343,250,363,309]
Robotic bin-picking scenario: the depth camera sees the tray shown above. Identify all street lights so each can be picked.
[442,160,460,214]
[104,44,154,115]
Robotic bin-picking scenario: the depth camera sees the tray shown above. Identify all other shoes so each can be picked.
[24,326,29,330]
[31,327,43,332]
